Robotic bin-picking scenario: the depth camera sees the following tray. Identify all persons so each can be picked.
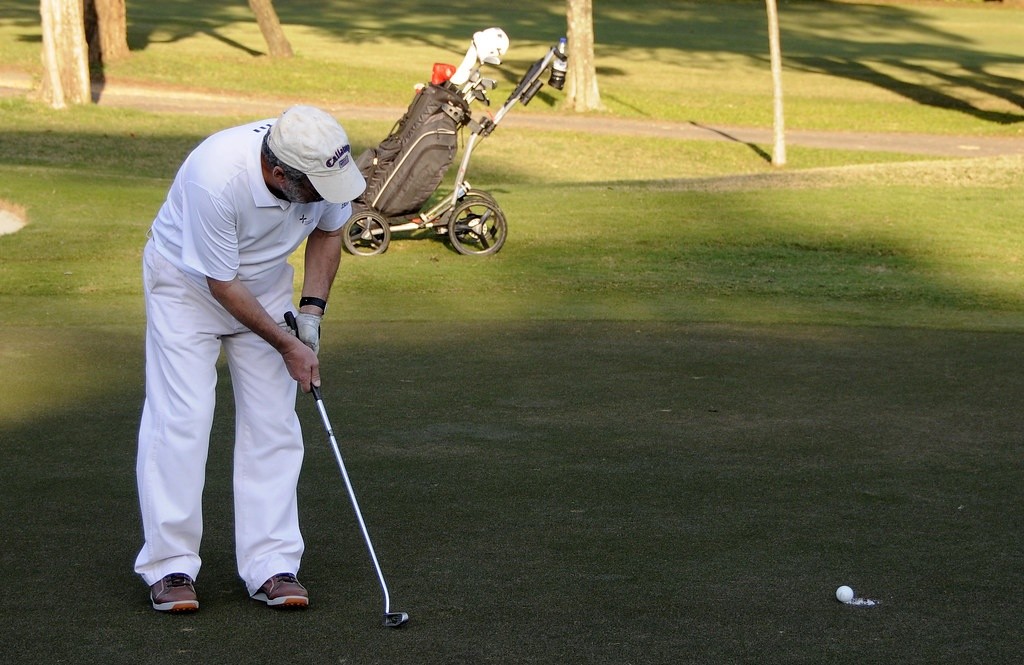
[133,103,368,611]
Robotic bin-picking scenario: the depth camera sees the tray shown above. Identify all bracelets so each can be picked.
[299,296,328,315]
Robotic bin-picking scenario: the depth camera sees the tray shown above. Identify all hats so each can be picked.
[265,104,367,203]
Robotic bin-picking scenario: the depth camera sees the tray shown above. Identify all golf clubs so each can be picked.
[449,54,506,107]
[283,310,410,629]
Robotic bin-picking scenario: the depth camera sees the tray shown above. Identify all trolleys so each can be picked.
[339,28,569,258]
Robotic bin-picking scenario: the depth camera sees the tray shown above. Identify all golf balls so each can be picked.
[836,586,855,604]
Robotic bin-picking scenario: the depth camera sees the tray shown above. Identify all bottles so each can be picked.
[552,38,567,79]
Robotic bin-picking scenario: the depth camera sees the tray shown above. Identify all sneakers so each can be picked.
[251,572,308,606]
[150,573,199,610]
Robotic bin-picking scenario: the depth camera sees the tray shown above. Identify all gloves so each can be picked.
[286,312,322,355]
